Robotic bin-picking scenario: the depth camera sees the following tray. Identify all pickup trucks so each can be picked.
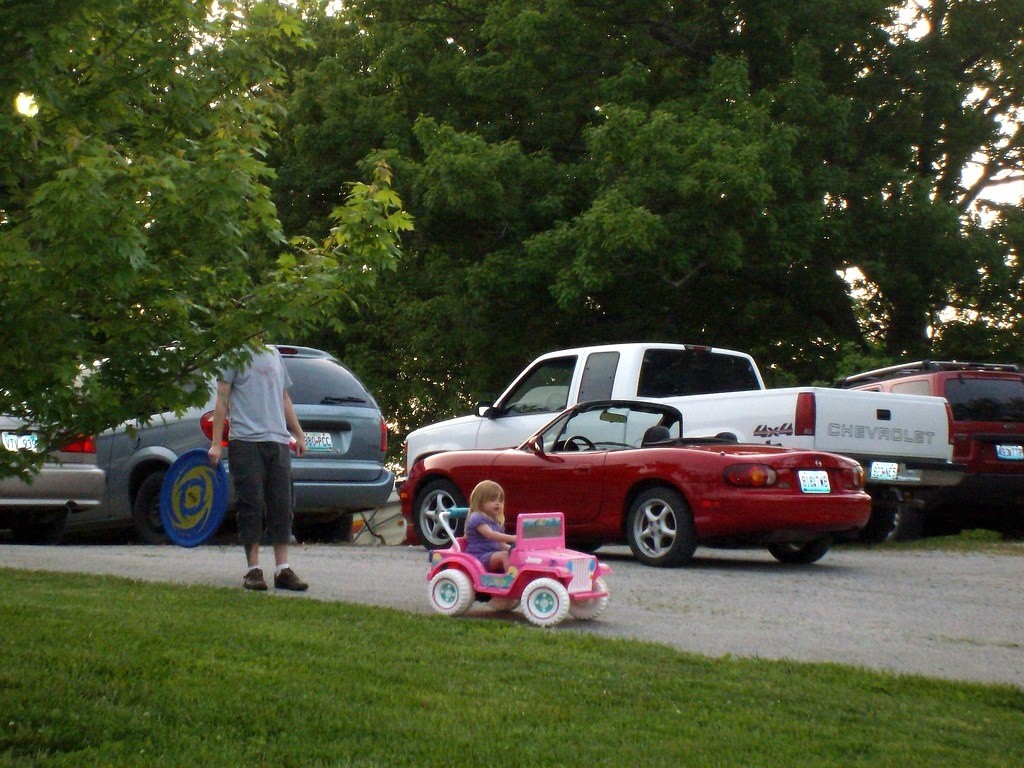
[400,341,955,552]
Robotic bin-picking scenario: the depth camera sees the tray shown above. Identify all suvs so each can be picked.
[831,357,1024,545]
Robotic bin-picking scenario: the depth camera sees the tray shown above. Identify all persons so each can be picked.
[464,480,517,573]
[208,298,308,590]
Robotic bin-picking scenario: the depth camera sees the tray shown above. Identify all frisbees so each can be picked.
[157,446,230,548]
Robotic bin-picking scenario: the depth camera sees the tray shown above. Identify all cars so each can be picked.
[66,340,393,544]
[0,391,106,543]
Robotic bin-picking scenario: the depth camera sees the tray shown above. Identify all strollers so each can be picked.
[426,502,613,628]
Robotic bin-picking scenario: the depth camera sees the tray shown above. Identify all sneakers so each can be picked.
[244,568,267,590]
[275,568,308,589]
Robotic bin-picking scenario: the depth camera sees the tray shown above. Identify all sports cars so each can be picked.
[398,394,874,569]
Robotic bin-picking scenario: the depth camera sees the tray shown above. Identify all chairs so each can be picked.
[641,426,669,449]
[716,431,738,441]
